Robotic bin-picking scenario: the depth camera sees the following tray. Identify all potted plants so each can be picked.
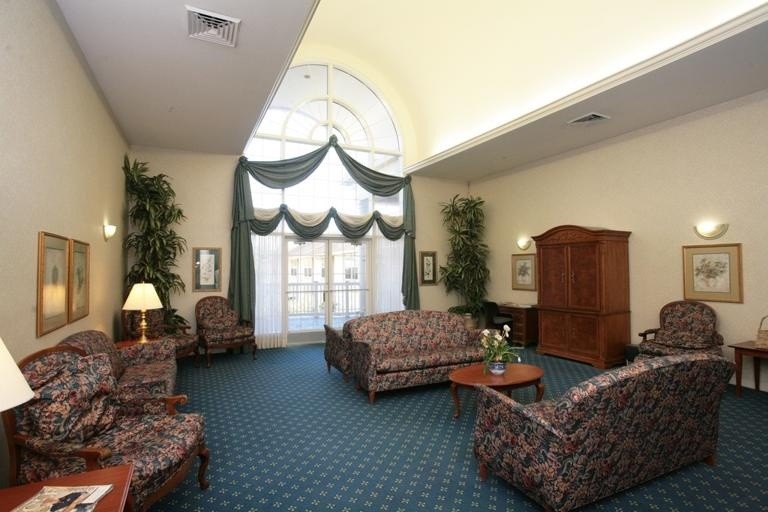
[435,190,494,332]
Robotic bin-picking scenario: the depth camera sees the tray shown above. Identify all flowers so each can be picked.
[477,322,524,377]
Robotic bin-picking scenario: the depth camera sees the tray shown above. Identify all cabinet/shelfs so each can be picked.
[485,300,539,348]
[529,223,632,315]
[534,310,631,370]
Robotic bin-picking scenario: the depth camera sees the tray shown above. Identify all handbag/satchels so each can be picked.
[755,316,768,349]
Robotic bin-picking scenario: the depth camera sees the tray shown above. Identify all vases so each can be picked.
[487,360,508,378]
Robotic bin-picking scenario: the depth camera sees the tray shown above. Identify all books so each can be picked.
[8,483,116,512]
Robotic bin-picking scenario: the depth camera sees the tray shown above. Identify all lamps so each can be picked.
[119,279,166,348]
[102,224,117,242]
[516,235,531,251]
[0,333,36,420]
[691,221,730,242]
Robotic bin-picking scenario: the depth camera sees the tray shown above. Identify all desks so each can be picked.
[1,461,138,512]
[726,339,768,400]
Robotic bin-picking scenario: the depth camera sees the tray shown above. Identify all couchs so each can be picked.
[471,351,739,511]
[347,308,502,405]
[55,327,179,396]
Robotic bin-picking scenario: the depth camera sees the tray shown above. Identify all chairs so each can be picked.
[192,295,259,368]
[122,301,202,374]
[0,342,214,512]
[322,317,354,384]
[637,299,726,358]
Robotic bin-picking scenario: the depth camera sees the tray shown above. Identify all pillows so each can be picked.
[16,351,132,446]
[652,329,717,350]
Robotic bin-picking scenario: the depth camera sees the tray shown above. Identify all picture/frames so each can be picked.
[418,250,438,286]
[191,246,223,294]
[67,237,91,325]
[680,242,746,305]
[34,227,71,339]
[510,252,538,291]
